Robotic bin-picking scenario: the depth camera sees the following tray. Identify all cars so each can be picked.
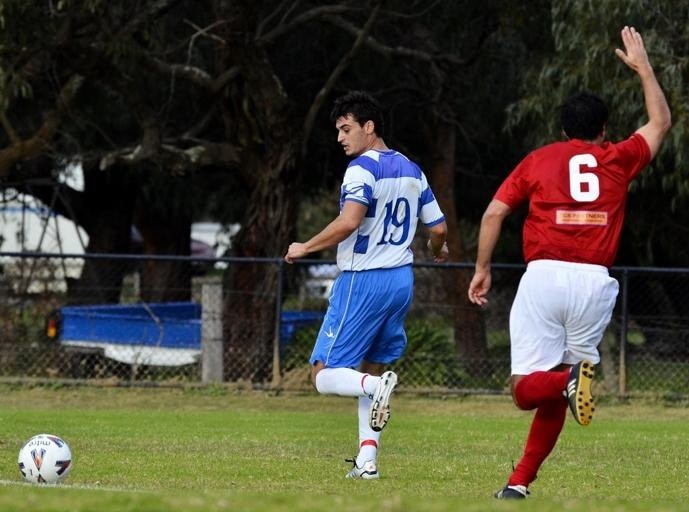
[121,220,244,275]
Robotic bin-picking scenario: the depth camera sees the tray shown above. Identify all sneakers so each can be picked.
[561,360,595,426]
[494,460,530,501]
[345,456,379,480]
[367,371,398,432]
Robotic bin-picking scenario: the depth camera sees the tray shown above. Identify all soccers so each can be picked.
[17,434,73,483]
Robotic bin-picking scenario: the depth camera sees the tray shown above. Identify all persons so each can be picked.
[462,23,681,500]
[282,93,455,481]
